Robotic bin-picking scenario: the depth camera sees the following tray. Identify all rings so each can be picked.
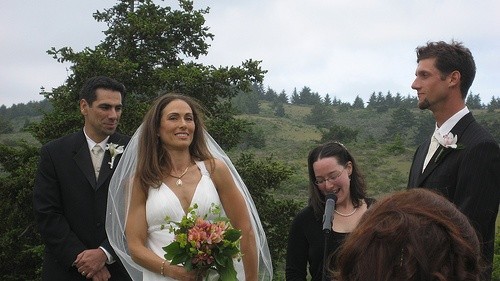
[82,271,85,275]
[197,274,200,278]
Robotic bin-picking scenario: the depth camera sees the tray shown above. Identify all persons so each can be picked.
[33,75,134,281]
[106,93,274,281]
[329,188,483,281]
[285,141,378,281]
[406,39,500,281]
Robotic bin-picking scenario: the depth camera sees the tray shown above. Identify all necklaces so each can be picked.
[170,162,191,186]
[334,208,357,217]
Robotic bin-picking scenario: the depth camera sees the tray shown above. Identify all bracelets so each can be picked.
[161,261,165,275]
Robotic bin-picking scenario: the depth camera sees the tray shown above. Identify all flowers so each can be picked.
[160,202,246,281]
[434,129,466,164]
[105,142,125,169]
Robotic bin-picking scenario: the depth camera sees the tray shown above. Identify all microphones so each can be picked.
[322,191,339,233]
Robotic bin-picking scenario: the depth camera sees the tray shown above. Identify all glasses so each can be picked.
[315,163,349,185]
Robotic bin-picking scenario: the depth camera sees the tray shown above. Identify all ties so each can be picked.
[93,146,102,159]
[422,129,439,173]
